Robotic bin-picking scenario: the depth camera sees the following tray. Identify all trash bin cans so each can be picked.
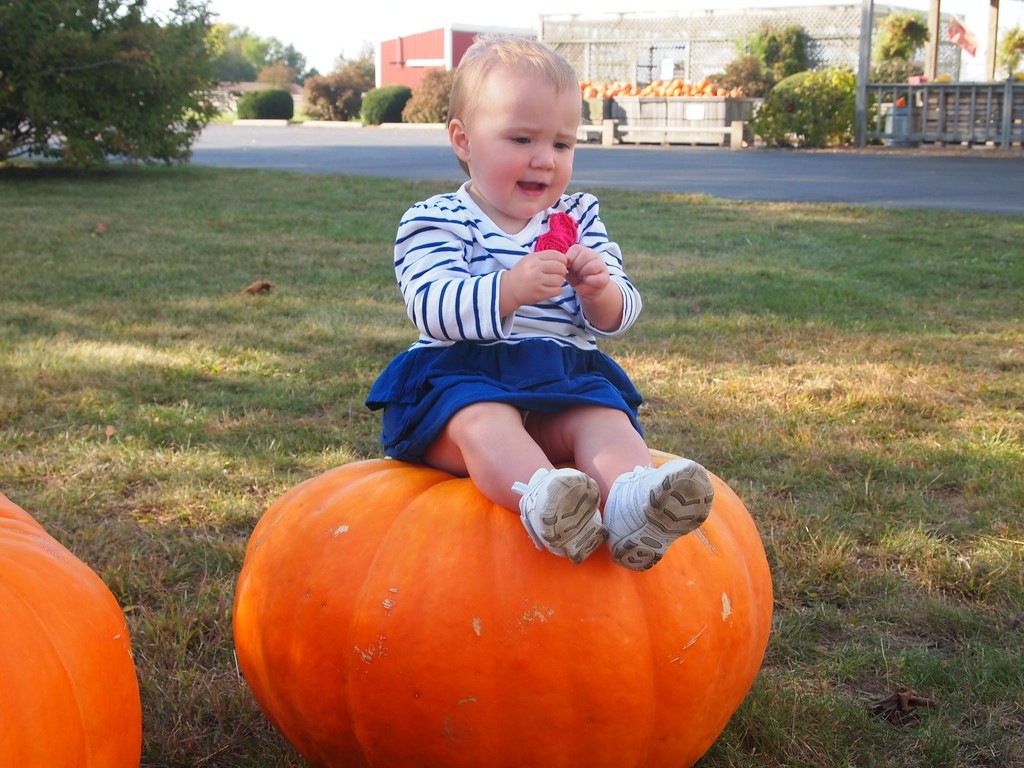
[886,106,909,146]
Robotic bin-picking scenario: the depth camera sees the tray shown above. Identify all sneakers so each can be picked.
[513,467,609,564]
[607,460,714,569]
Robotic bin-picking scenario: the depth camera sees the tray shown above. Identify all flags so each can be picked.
[948,13,979,54]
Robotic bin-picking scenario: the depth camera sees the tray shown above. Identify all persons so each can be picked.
[364,33,714,572]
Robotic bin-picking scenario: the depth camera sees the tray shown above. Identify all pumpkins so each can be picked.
[232,454,774,768]
[576,79,742,96]
[1,491,142,768]
